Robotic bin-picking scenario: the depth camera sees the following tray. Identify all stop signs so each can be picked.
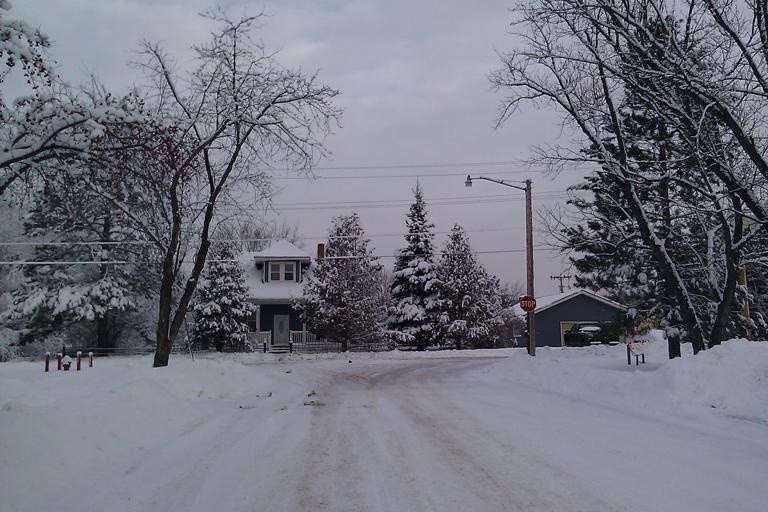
[520,295,536,312]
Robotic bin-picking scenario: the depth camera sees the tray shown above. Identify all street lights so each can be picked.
[464,174,536,356]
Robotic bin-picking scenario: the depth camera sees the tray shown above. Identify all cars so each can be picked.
[564,321,609,347]
